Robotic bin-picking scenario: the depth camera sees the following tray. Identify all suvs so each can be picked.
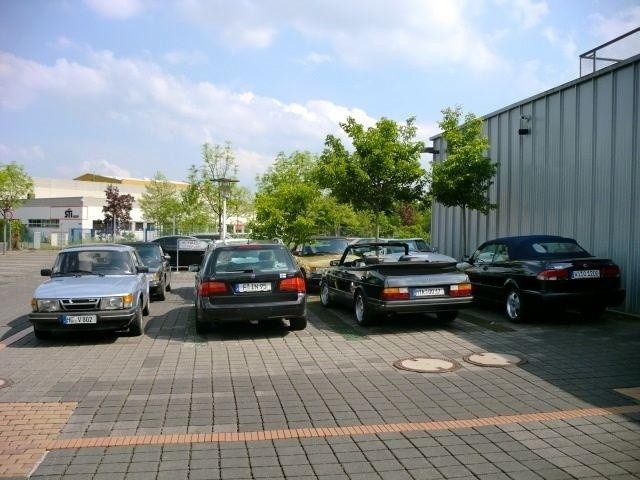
[188,237,308,335]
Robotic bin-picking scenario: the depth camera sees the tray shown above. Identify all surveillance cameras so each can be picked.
[520,112,530,122]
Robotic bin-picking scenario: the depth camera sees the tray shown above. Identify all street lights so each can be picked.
[2,208,10,254]
[210,178,240,239]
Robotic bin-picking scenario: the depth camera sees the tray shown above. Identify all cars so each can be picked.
[27,243,150,340]
[150,235,208,266]
[108,241,172,301]
[344,238,457,264]
[286,235,366,294]
[318,241,476,326]
[455,234,625,324]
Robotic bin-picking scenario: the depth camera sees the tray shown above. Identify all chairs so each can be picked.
[216,252,236,272]
[366,256,379,264]
[256,251,275,269]
[110,252,132,273]
[74,260,93,272]
[398,254,428,261]
